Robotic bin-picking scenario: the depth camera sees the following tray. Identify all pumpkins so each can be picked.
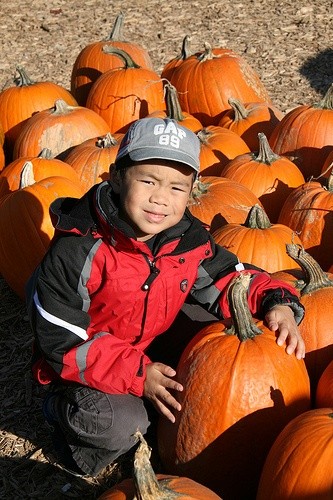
[0,16,333,500]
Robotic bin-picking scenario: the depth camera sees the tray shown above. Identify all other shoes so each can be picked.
[43,394,103,478]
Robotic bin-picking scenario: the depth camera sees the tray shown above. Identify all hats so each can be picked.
[115,117,201,181]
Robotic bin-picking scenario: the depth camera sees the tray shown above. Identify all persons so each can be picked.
[25,117,306,489]
